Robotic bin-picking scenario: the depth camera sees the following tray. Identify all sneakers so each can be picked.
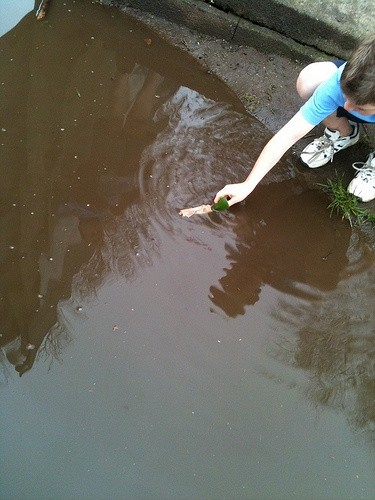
[347,152,375,203]
[299,120,360,169]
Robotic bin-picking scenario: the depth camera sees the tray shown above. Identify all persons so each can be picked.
[213,36,375,207]
